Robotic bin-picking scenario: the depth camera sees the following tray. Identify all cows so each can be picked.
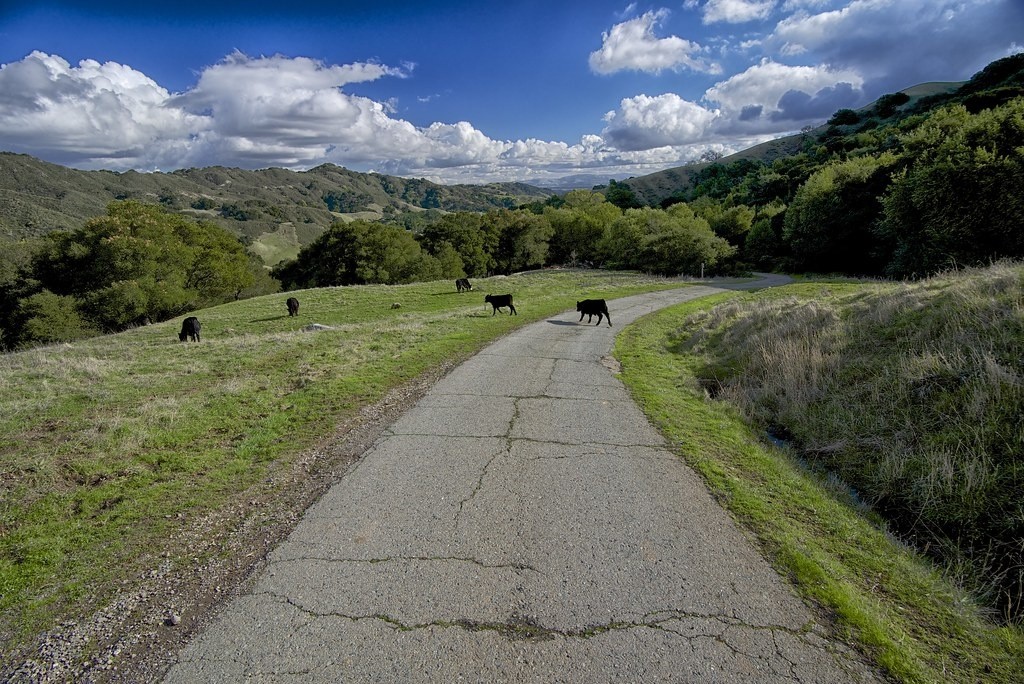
[577,299,613,327]
[484,292,518,317]
[178,316,201,342]
[286,297,300,317]
[455,278,472,293]
